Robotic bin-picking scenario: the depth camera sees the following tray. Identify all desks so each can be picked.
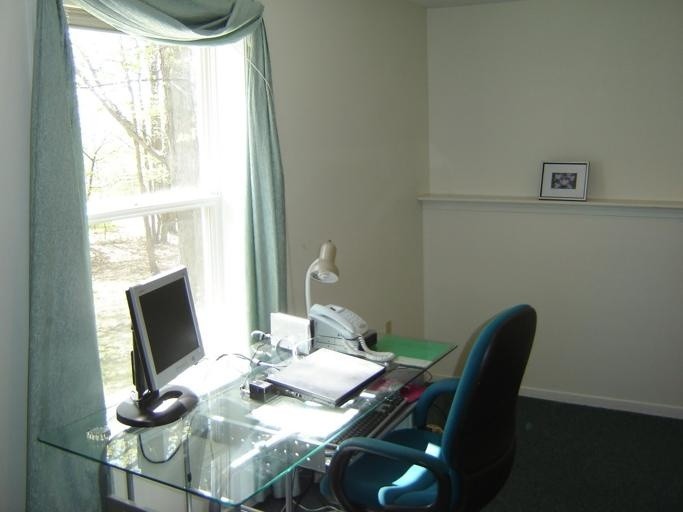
[35,335,460,512]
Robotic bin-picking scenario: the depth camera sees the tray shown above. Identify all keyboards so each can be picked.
[325,392,408,454]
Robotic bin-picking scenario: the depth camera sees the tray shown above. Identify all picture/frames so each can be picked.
[538,161,590,201]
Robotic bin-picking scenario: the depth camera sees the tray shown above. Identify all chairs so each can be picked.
[317,303,537,512]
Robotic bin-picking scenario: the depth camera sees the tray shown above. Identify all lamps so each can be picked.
[304,237,340,315]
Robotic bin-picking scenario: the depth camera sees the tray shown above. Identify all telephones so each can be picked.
[309,304,368,340]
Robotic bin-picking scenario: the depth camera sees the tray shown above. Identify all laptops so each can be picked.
[266,347,386,409]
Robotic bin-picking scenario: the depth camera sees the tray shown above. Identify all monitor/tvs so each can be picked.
[116,265,205,427]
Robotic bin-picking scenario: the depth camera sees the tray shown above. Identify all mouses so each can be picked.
[390,383,412,395]
